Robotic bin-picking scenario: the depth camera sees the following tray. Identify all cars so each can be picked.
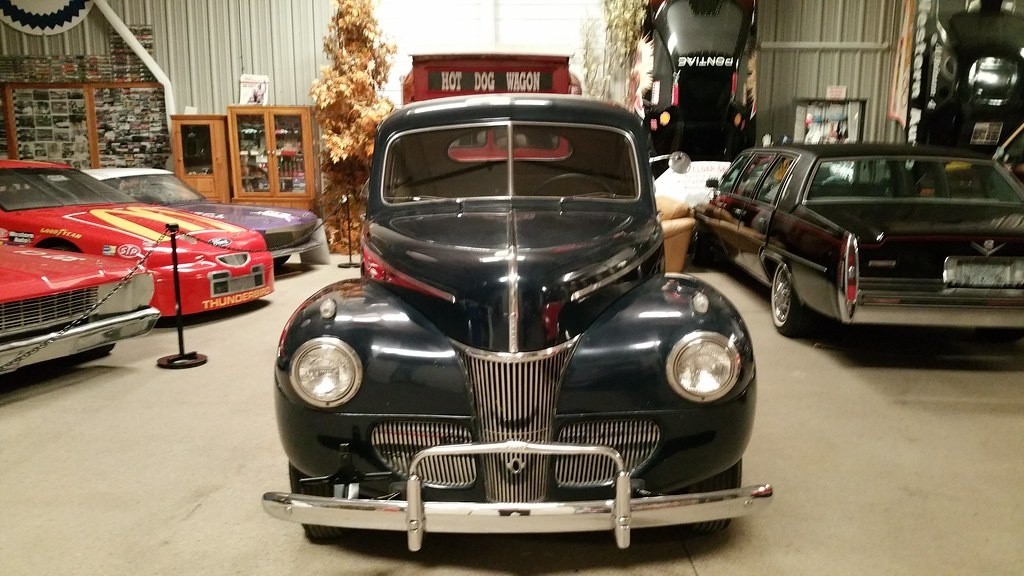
[1,159,323,376]
[260,92,773,550]
[687,144,1024,336]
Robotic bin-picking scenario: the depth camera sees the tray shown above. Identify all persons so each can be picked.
[16,100,87,167]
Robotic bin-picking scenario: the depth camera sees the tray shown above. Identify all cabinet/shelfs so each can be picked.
[171,104,323,220]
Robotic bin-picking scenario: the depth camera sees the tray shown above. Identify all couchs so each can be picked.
[657,196,697,275]
[693,202,785,255]
